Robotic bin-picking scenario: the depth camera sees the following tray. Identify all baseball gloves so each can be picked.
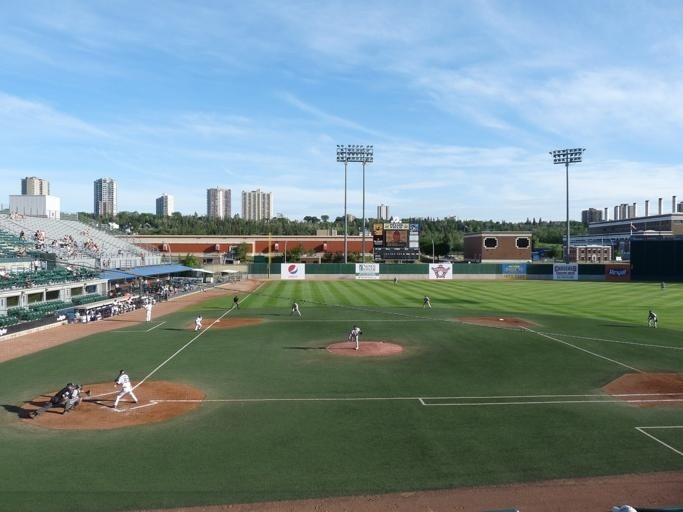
[86,390,91,395]
[358,331,362,334]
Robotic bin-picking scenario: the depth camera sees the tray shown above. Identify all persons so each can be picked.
[6,212,27,219]
[394,276,398,287]
[61,384,91,416]
[1,230,203,324]
[112,370,138,409]
[348,325,362,350]
[389,231,405,247]
[230,295,240,310]
[648,311,657,328]
[194,316,203,331]
[290,301,302,317]
[28,382,74,419]
[424,296,432,309]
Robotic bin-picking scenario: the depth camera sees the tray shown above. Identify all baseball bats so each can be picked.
[115,373,123,381]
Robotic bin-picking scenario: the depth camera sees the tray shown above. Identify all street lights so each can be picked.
[337,144,374,263]
[265,218,271,279]
[549,147,586,254]
